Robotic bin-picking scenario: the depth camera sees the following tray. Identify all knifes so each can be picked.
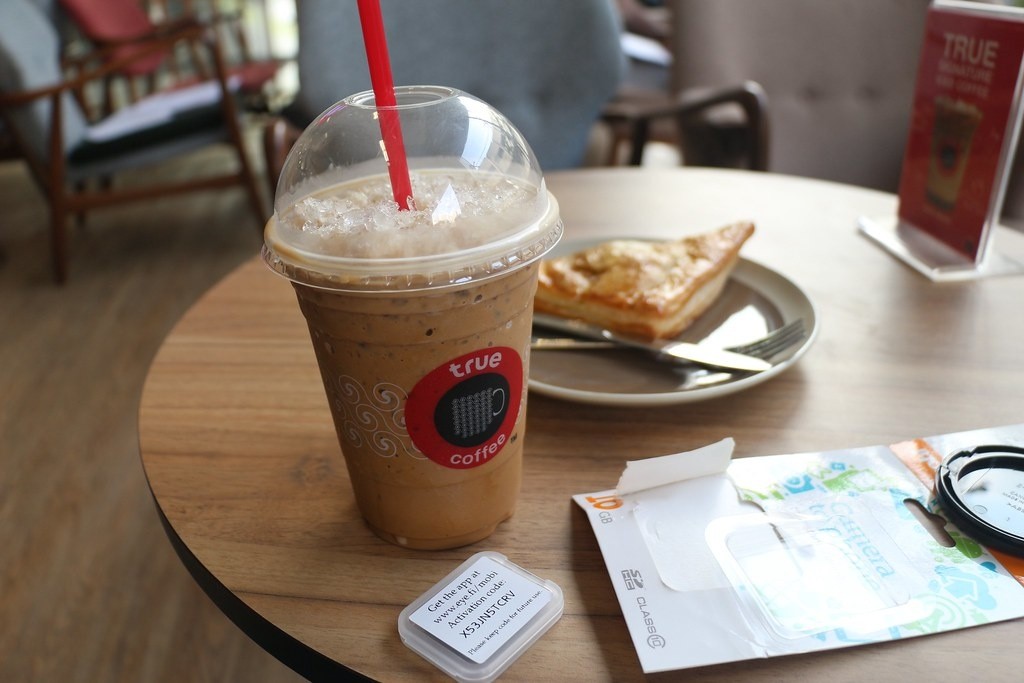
[532,313,773,372]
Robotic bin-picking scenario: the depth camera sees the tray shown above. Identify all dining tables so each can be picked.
[136,165,1023,683]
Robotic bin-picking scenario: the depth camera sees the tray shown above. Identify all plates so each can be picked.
[526,233,819,407]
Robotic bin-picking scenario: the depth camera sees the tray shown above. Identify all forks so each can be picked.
[533,317,804,364]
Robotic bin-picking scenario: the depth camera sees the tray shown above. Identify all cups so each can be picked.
[260,87,566,551]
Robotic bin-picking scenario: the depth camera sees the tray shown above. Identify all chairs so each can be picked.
[595,5,776,173]
[1,2,278,283]
[298,0,627,187]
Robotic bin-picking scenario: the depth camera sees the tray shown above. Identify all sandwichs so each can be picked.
[534,220,756,341]
